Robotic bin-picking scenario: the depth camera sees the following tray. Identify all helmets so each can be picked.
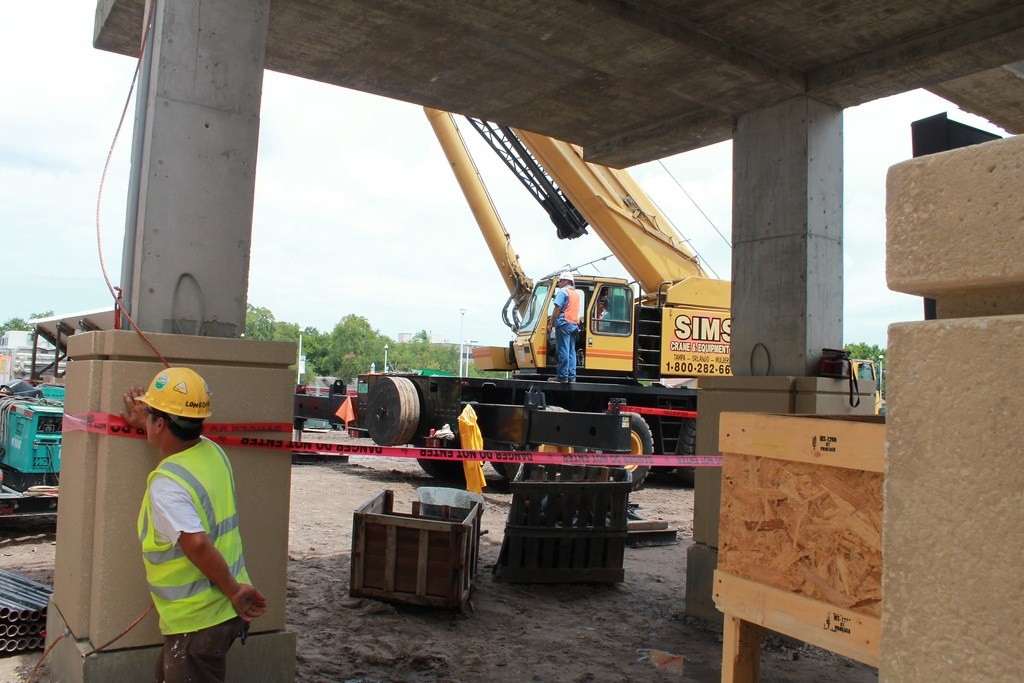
[559,272,574,282]
[134,368,212,419]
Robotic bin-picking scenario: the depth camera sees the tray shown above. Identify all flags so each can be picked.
[336,397,356,431]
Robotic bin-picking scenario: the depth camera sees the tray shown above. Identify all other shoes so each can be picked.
[549,377,576,383]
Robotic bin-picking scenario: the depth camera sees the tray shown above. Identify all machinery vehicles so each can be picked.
[293,104,736,494]
[0,375,76,529]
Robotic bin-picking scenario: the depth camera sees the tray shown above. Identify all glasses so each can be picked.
[144,407,167,420]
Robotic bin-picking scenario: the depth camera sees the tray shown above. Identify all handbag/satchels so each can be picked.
[820,348,860,407]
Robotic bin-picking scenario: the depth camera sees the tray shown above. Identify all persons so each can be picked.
[547,273,580,383]
[576,296,610,366]
[120,368,268,683]
